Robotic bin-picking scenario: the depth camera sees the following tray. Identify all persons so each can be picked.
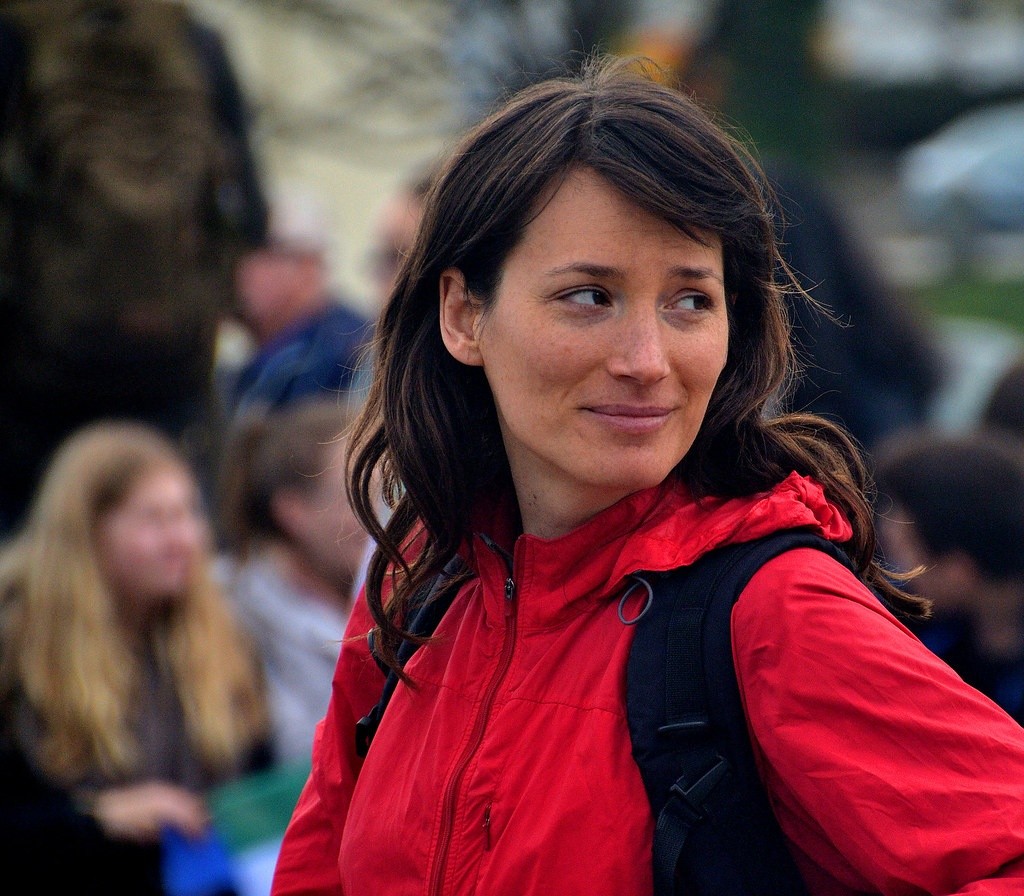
[459,0,1024,724]
[270,53,1024,896]
[0,0,387,896]
